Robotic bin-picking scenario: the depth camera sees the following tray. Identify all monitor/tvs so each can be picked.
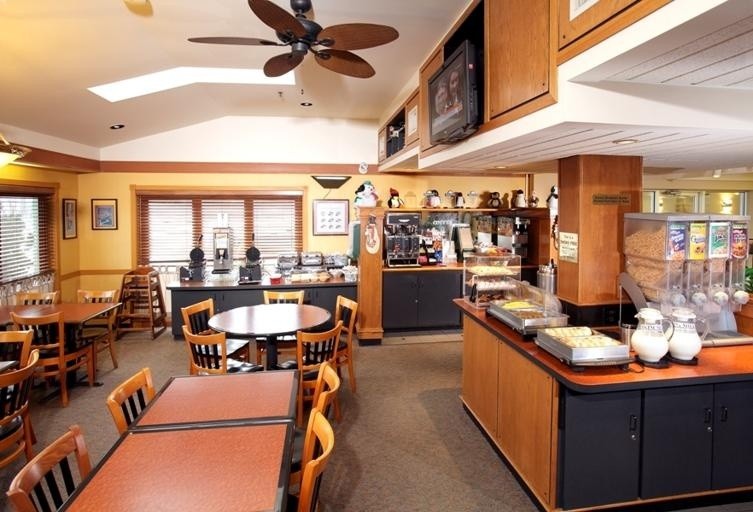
[426,42,480,146]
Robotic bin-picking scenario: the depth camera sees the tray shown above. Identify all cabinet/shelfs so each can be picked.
[263,286,358,338]
[382,272,461,331]
[169,289,263,341]
[461,314,554,506]
[641,380,753,501]
[559,389,642,509]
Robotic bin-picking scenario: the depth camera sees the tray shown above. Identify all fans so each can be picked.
[187,0,399,80]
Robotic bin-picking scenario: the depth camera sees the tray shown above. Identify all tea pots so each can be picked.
[666,307,709,366]
[631,308,675,369]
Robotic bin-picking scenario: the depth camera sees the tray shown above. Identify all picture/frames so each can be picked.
[62,198,78,241]
[91,198,119,231]
[311,199,350,237]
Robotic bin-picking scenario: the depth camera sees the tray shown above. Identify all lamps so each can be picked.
[0,132,32,168]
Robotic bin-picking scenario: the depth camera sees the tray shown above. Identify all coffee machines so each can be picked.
[212,228,234,274]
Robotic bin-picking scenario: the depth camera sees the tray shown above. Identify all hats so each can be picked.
[365,180,370,185]
[356,185,364,193]
[391,188,397,194]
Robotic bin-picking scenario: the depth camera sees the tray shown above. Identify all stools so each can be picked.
[112,266,167,341]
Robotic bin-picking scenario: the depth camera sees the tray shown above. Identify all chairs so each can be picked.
[284,408,336,512]
[5,424,93,512]
[277,319,343,429]
[0,348,42,469]
[105,366,157,437]
[77,289,122,382]
[301,294,358,393]
[182,324,264,375]
[257,289,305,365]
[1,324,37,446]
[288,360,341,510]
[9,310,94,410]
[180,297,250,359]
[15,291,61,388]
